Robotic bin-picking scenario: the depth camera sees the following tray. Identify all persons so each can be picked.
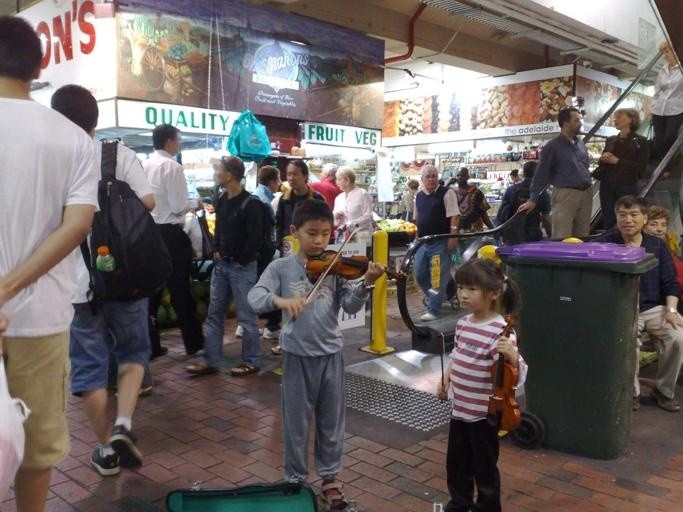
[0,11,103,512]
[114,123,375,400]
[494,159,551,244]
[404,165,491,323]
[436,258,529,512]
[642,204,683,313]
[50,84,156,479]
[246,199,384,512]
[648,40,682,182]
[585,190,683,415]
[516,105,595,240]
[593,105,651,232]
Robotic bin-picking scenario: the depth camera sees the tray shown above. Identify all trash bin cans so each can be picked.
[494,237,659,460]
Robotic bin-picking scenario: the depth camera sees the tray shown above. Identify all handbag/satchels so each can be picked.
[591,165,602,180]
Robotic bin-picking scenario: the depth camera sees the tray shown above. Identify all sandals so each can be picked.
[320,482,348,512]
[231,365,258,376]
[187,363,215,373]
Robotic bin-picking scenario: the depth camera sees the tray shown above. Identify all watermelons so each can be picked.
[156,307,167,328]
[228,303,238,321]
[161,290,174,309]
[191,282,211,298]
[195,303,208,319]
[167,307,178,324]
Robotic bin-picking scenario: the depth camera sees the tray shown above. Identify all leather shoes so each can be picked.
[633,395,641,410]
[151,346,168,361]
[653,387,681,412]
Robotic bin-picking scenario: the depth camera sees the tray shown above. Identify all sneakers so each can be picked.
[90,448,120,476]
[421,313,440,320]
[110,424,143,467]
[235,325,282,354]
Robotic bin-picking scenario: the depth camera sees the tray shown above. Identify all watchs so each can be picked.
[664,307,677,313]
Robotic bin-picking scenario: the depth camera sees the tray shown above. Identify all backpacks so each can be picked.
[79,138,175,303]
[241,195,276,256]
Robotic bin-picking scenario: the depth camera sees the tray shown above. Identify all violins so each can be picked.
[304,249,410,281]
[485,314,522,433]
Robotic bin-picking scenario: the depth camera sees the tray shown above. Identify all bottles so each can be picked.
[93,246,117,272]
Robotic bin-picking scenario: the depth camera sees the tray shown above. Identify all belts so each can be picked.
[221,256,235,263]
[561,184,591,191]
